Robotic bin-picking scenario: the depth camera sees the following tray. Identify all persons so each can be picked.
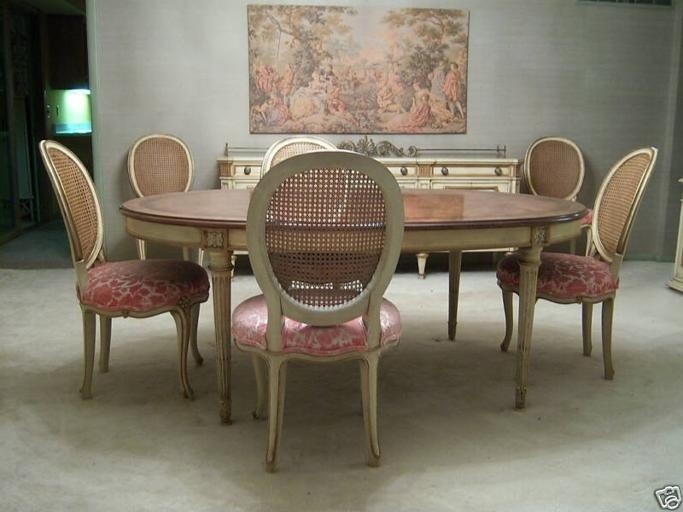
[390,81,434,131]
[425,58,449,122]
[443,62,465,120]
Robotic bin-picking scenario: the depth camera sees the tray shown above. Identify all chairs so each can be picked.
[40,133,660,471]
